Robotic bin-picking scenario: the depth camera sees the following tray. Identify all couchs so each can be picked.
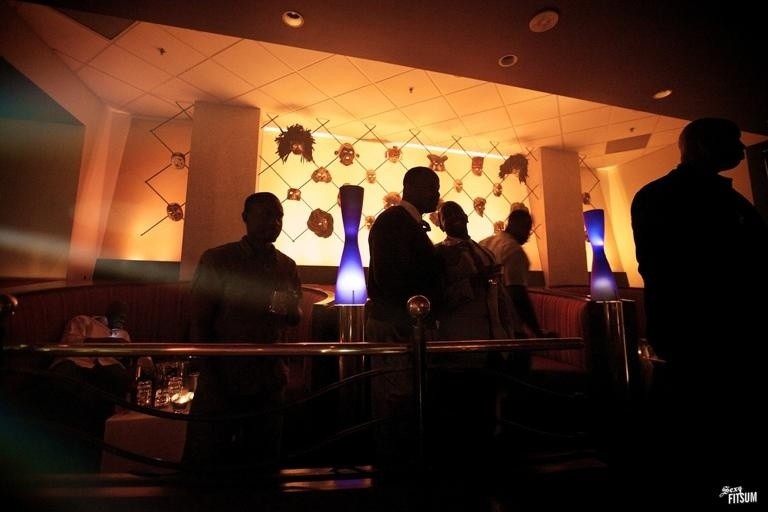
[0,281,340,407]
[527,287,611,389]
[544,283,648,399]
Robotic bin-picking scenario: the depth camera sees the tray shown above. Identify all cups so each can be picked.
[268,291,290,315]
[137,381,152,405]
[176,361,184,377]
[155,389,170,406]
[485,264,498,288]
[167,377,183,396]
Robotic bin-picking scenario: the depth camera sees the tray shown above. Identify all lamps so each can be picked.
[335,184,368,307]
[583,208,619,301]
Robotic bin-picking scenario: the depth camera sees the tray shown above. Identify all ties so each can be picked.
[467,244,484,269]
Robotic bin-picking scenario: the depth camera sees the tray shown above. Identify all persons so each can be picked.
[477,208,560,445]
[364,165,449,466]
[432,201,509,444]
[45,299,132,473]
[631,116,768,511]
[180,192,302,511]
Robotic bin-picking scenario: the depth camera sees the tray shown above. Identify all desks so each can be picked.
[101,410,188,475]
